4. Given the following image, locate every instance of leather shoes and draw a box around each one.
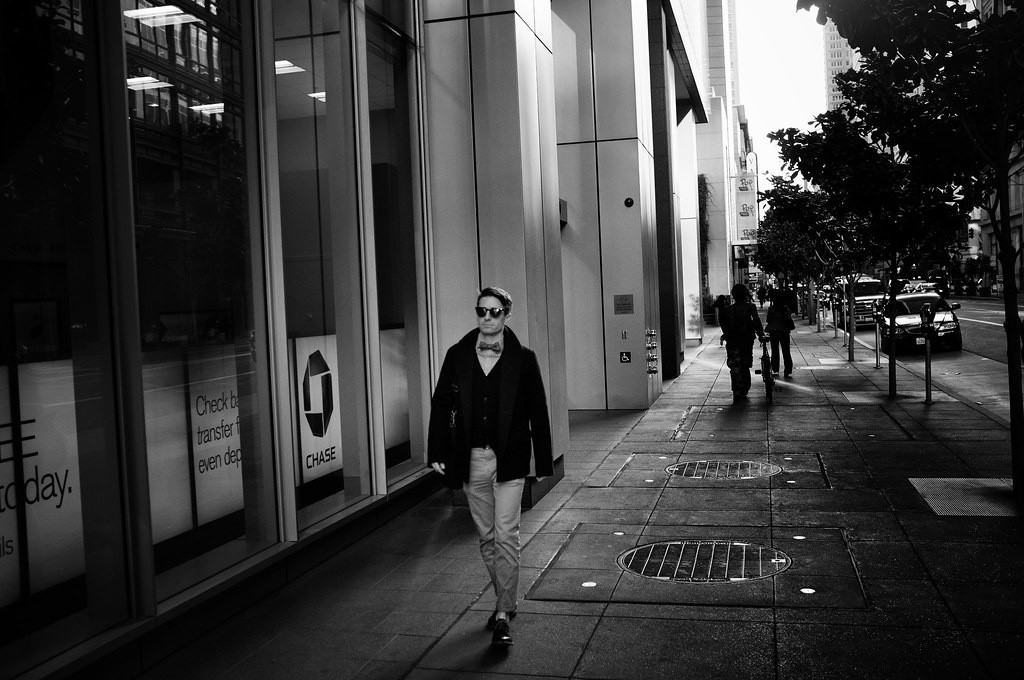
[486,609,516,630]
[492,619,513,644]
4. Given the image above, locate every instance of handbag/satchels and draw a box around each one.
[765,322,775,334]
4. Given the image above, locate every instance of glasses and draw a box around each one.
[475,306,505,318]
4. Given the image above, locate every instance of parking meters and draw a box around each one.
[821,293,827,331]
[871,299,882,369]
[831,292,839,339]
[918,302,937,405]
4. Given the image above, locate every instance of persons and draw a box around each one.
[712,282,796,400]
[427,285,555,648]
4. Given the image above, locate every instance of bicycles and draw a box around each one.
[757,332,780,410]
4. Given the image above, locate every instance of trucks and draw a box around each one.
[813,276,885,331]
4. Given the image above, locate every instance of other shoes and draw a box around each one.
[771,369,779,378]
[734,394,748,408]
[784,370,792,378]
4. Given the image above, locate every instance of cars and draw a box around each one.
[879,293,962,357]
[889,279,947,296]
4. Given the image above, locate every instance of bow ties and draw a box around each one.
[480,341,501,351]
